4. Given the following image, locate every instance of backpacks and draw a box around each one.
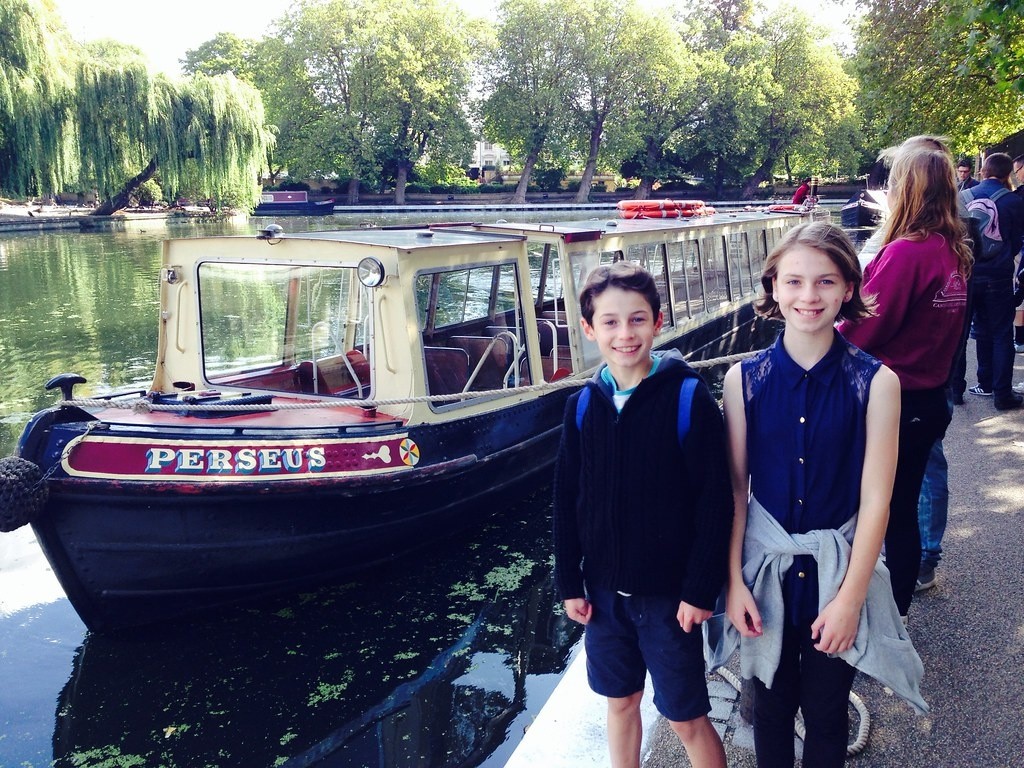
[961,188,1012,261]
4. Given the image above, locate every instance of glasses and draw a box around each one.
[1014,165,1022,175]
[958,170,969,173]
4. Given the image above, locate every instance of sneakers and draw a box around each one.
[914,569,937,593]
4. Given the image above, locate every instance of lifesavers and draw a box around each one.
[616,198,717,219]
[768,205,798,211]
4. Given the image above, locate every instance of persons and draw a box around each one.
[831,148,976,634]
[880,135,966,594]
[553,261,739,765]
[715,217,904,768]
[790,177,819,211]
[944,151,1024,414]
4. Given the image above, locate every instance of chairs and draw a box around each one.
[295,251,766,408]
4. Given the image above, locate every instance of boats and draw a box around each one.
[841,172,890,241]
[0,190,840,637]
